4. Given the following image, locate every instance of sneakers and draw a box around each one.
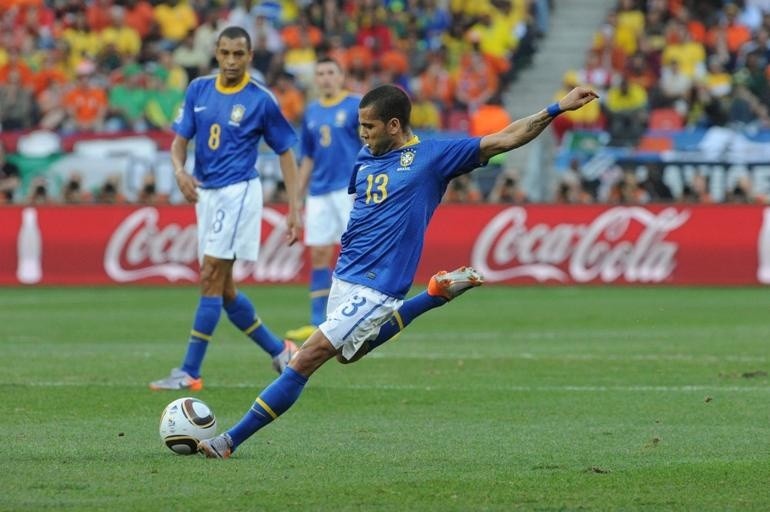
[149,368,202,390]
[428,266,485,301]
[273,339,300,374]
[198,434,234,458]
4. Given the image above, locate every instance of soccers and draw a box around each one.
[159,398,217,454]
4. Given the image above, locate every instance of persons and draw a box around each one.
[146,25,303,394]
[278,56,363,343]
[195,84,598,460]
[0,1,770,204]
